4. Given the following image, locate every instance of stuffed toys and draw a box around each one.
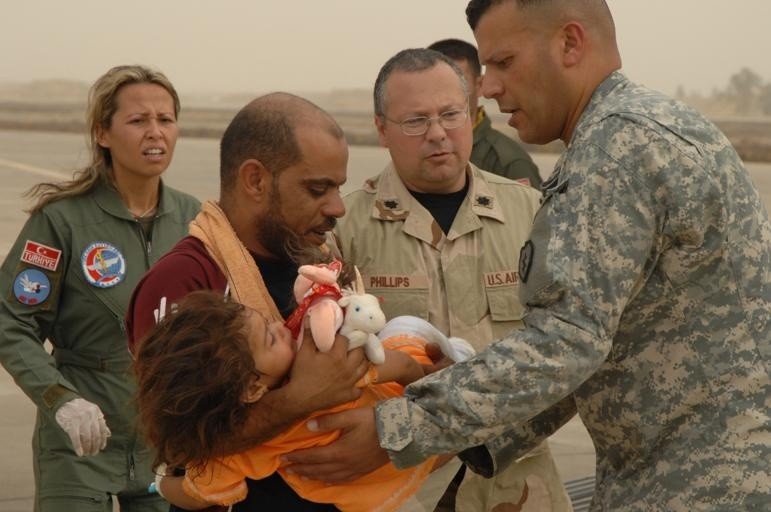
[288,258,347,355]
[336,263,389,366]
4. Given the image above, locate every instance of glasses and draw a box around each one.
[380,96,470,136]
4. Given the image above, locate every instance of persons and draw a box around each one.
[1,62,205,512]
[279,0,769,512]
[133,285,531,510]
[124,90,470,512]
[312,46,578,511]
[428,35,547,194]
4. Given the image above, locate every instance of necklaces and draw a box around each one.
[125,196,158,218]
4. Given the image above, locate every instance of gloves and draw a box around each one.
[55,398,112,456]
